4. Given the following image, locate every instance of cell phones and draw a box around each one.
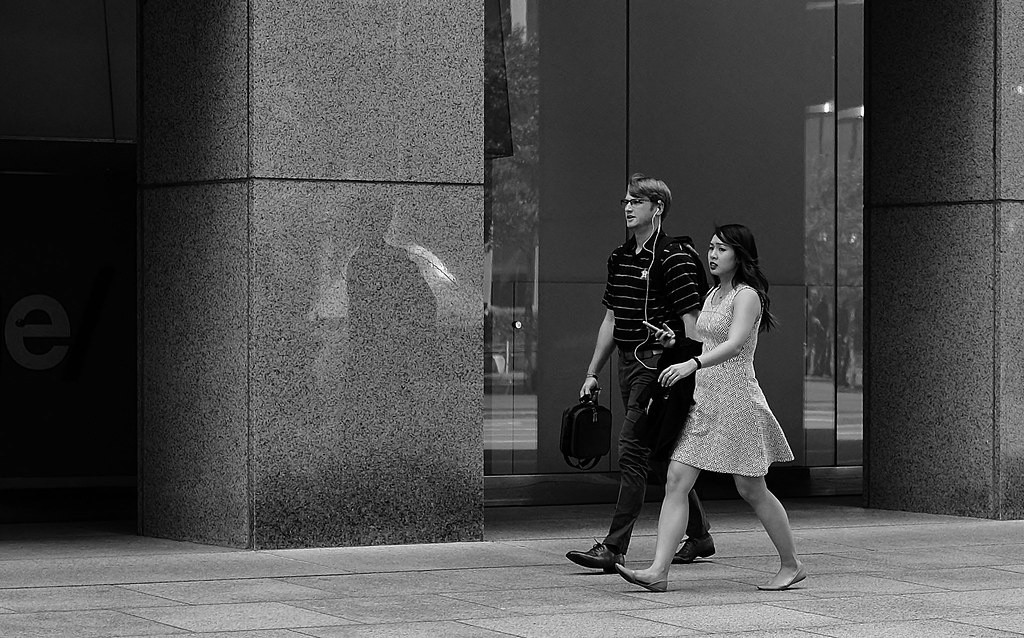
[643,321,671,341]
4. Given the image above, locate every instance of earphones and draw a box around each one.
[655,208,660,213]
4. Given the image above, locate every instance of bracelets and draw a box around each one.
[692,357,701,370]
[586,373,598,380]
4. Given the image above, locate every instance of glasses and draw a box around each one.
[620,199,650,208]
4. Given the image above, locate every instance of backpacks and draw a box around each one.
[655,236,709,310]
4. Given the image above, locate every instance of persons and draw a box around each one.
[615,223,807,592]
[565,172,715,574]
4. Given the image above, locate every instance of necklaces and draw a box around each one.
[719,289,732,298]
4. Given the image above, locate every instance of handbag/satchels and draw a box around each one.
[559,396,612,470]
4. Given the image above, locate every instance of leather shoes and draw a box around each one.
[672,535,715,563]
[566,538,625,573]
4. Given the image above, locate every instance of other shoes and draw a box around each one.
[757,565,807,590]
[615,562,668,592]
